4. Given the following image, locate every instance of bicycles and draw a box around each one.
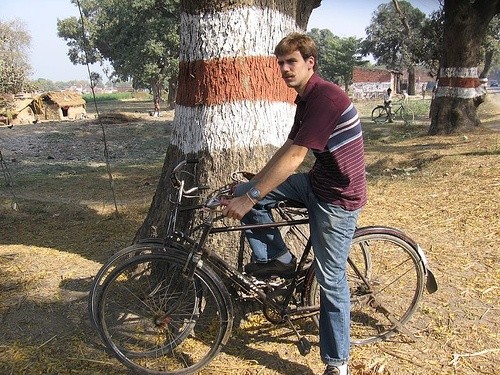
[87,159,372,358]
[371,96,415,125]
[96,188,438,372]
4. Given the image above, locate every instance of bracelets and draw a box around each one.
[245,192,256,206]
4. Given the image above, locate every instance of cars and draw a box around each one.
[491,83,498,87]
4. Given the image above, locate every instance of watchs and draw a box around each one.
[248,186,262,202]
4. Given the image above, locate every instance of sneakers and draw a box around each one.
[322,363,351,375]
[245,249,297,278]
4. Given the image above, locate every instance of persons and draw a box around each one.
[218,33,366,375]
[153,95,160,117]
[422,84,426,100]
[384,88,395,123]
